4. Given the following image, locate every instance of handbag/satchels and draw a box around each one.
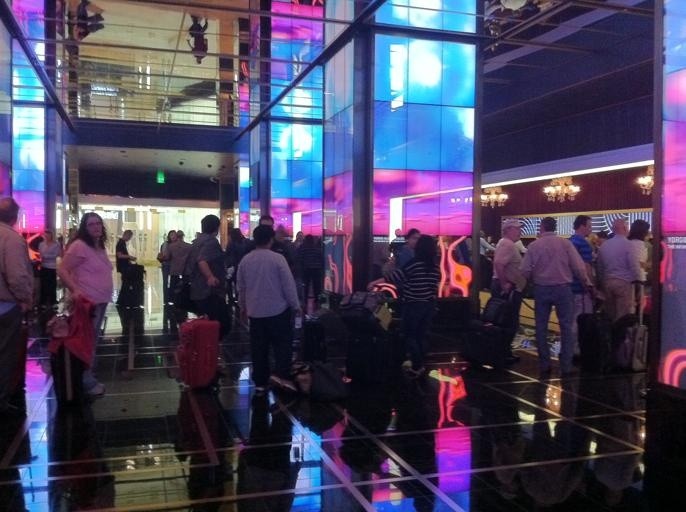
[340,290,382,320]
[48,314,75,338]
[201,295,232,338]
[172,277,191,309]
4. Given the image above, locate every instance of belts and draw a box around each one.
[538,283,569,287]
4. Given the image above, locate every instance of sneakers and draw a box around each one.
[83,383,104,395]
[503,354,519,363]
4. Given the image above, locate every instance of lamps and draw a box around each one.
[634,163,656,196]
[480,187,508,208]
[542,177,580,201]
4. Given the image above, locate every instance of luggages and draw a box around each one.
[178,318,220,390]
[281,314,326,366]
[616,280,649,371]
[577,284,609,362]
[122,265,144,307]
[52,296,95,404]
[468,290,515,381]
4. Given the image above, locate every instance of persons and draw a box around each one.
[159,230,193,340]
[37,229,59,309]
[192,215,233,342]
[246,215,293,269]
[490,218,528,366]
[275,226,326,314]
[584,230,616,258]
[237,391,305,512]
[187,15,208,64]
[0,198,37,412]
[629,219,650,323]
[463,374,643,512]
[596,219,647,323]
[116,230,137,304]
[57,213,113,396]
[226,227,255,263]
[395,229,421,269]
[325,236,338,271]
[479,230,496,289]
[156,230,178,304]
[561,215,594,358]
[366,235,441,378]
[236,225,304,391]
[338,376,441,512]
[519,217,594,375]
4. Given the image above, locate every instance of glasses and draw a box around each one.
[82,221,103,226]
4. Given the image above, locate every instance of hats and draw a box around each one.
[502,218,524,229]
